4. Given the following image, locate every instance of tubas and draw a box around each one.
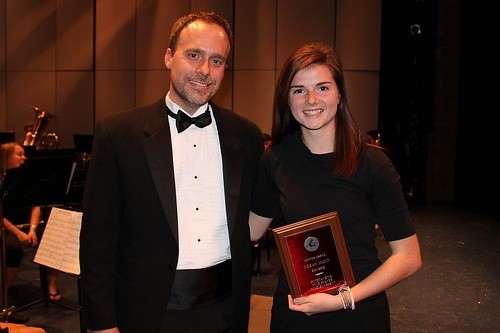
[22,104,59,151]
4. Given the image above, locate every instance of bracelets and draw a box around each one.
[30,225,38,230]
[337,285,355,310]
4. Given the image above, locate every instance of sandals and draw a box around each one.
[49,289,62,301]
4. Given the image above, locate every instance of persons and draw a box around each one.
[0,142,61,306]
[79,12,378,333]
[261,131,273,153]
[248,40,423,333]
[364,129,388,153]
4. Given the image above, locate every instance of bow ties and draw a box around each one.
[166,106,212,134]
